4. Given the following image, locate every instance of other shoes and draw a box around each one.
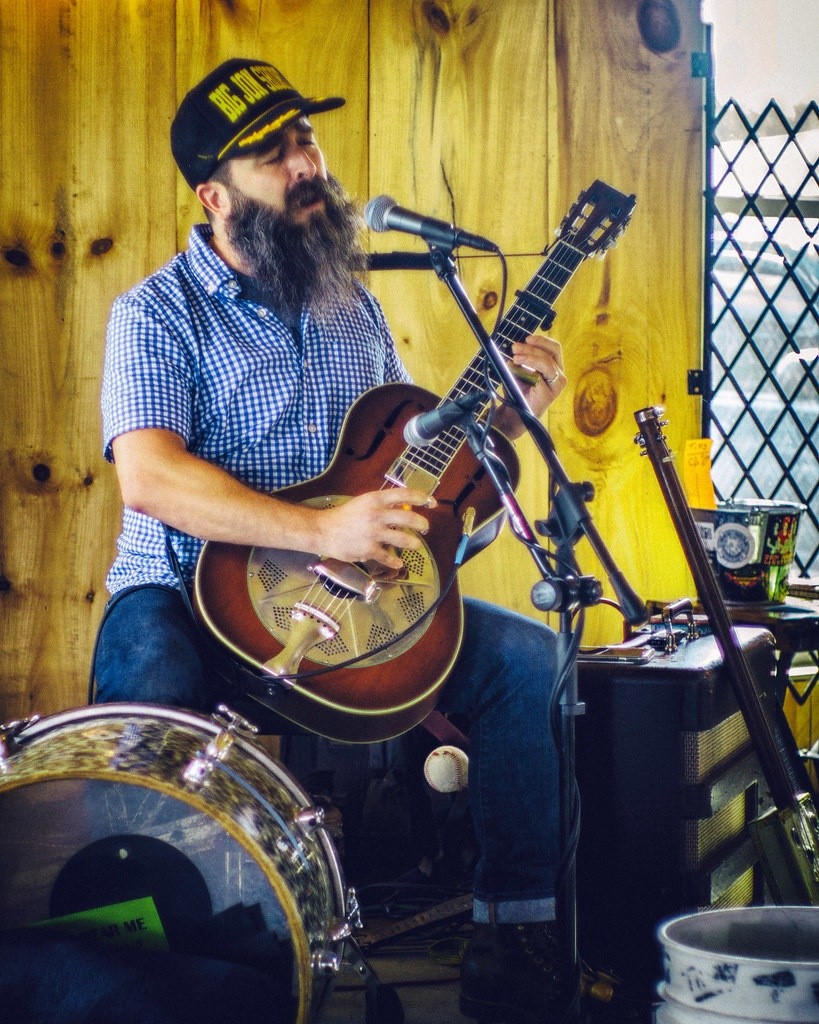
[457,922,635,1022]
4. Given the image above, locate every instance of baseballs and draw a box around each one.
[423,745,469,794]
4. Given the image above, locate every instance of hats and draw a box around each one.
[170,58,346,193]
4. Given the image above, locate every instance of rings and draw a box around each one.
[547,372,559,383]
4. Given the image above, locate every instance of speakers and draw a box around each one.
[574,622,772,966]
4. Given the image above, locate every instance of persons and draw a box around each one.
[93,58,653,1024]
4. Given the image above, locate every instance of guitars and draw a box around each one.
[190,180,639,743]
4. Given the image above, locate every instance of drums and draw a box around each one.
[1,704,352,1024]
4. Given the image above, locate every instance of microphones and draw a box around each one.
[403,388,484,447]
[363,194,499,254]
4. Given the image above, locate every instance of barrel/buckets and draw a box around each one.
[690,498,808,606]
[655,906,819,1024]
